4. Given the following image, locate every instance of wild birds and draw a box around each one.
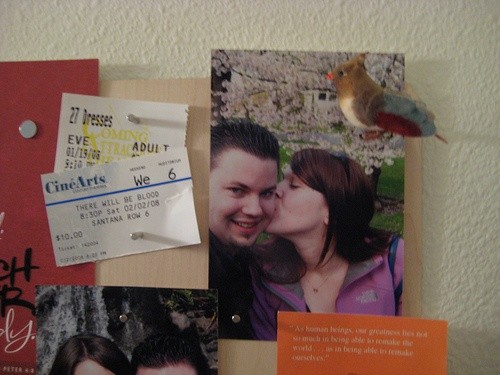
[325,51,437,141]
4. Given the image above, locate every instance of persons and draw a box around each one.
[248,145,403,340]
[47,333,133,375]
[133,326,216,375]
[24,120,280,375]
